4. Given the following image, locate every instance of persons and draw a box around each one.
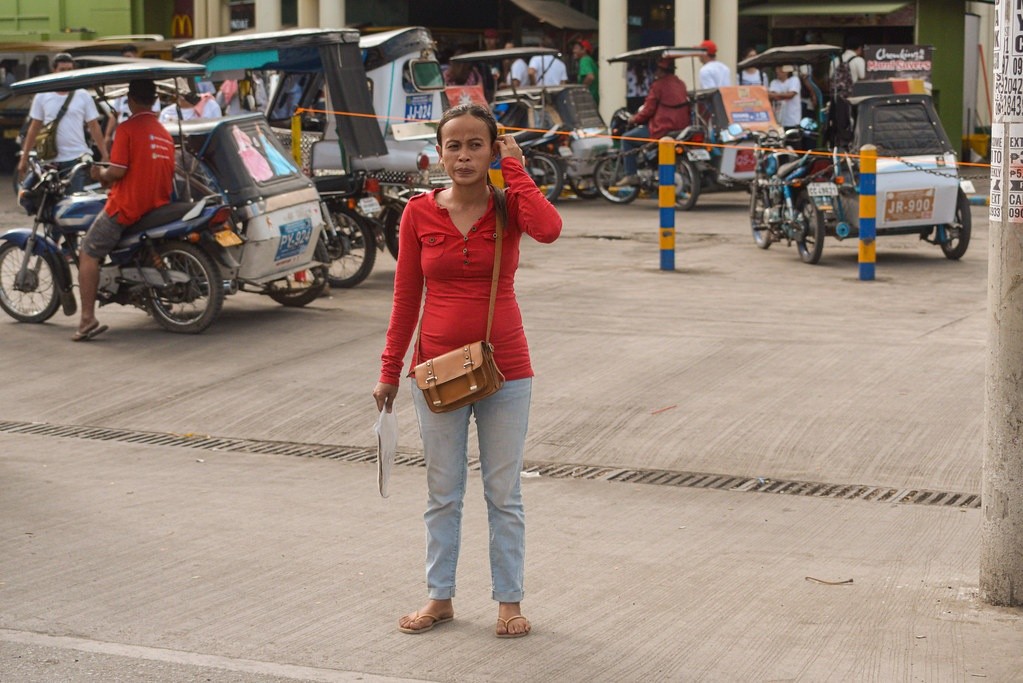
[373,104,564,639]
[444,38,602,107]
[69,77,177,344]
[18,55,117,195]
[617,59,693,189]
[699,41,867,149]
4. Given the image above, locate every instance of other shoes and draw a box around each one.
[615,176,641,186]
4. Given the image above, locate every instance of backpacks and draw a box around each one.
[829,54,858,102]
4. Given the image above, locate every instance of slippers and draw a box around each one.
[398,609,453,634]
[71,325,107,342]
[496,615,529,637]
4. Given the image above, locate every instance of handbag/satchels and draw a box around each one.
[35,129,58,161]
[414,341,506,413]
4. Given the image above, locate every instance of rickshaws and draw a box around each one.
[0,33,166,175]
[169,26,457,289]
[0,57,334,334]
[438,45,615,204]
[736,42,972,265]
[594,44,787,210]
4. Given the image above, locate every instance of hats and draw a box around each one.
[700,40,717,56]
[484,29,498,38]
[576,38,592,53]
